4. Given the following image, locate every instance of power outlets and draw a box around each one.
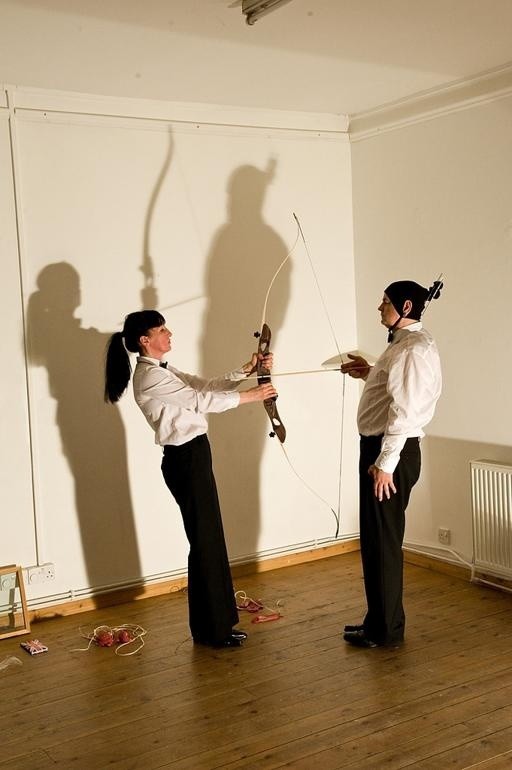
[40,563,56,585]
[438,528,451,545]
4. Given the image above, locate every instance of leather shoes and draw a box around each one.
[205,637,241,646]
[230,631,246,641]
[342,624,364,632]
[343,630,378,647]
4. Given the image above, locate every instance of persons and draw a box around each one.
[340,281,443,648]
[103,310,278,646]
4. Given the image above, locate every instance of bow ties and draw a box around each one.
[387,331,394,343]
[160,361,169,370]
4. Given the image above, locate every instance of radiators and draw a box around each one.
[468,458,512,581]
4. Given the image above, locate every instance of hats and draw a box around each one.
[385,281,443,320]
